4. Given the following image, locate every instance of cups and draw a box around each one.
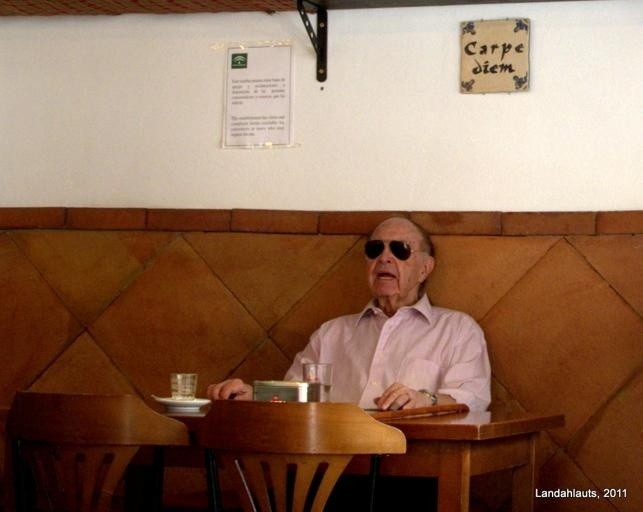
[303,363,334,403]
[170,372,198,401]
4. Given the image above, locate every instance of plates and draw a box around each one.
[150,394,212,413]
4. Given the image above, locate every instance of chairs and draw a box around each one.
[9,390,407,511]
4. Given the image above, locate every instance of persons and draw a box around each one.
[207,216,492,512]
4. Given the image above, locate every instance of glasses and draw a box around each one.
[364,239,430,261]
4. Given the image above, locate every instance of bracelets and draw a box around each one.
[419,389,438,405]
